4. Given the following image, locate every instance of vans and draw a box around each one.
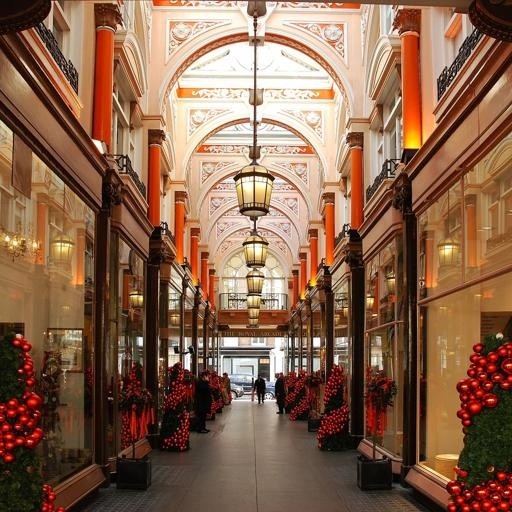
[227,373,258,395]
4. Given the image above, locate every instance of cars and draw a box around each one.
[264,381,278,400]
[230,382,244,400]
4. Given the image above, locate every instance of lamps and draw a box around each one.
[235,0,281,217]
[169,312,181,328]
[384,255,398,297]
[241,216,270,327]
[333,258,379,328]
[0,225,40,266]
[436,185,464,271]
[128,289,145,312]
[51,182,75,270]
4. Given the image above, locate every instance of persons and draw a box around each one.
[222,372,232,403]
[280,372,285,382]
[252,373,267,405]
[273,373,286,415]
[194,372,210,434]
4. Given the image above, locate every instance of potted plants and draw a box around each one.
[284,367,324,434]
[319,365,350,451]
[112,365,162,497]
[351,360,402,492]
[144,362,224,452]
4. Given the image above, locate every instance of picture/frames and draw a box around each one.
[43,325,83,376]
